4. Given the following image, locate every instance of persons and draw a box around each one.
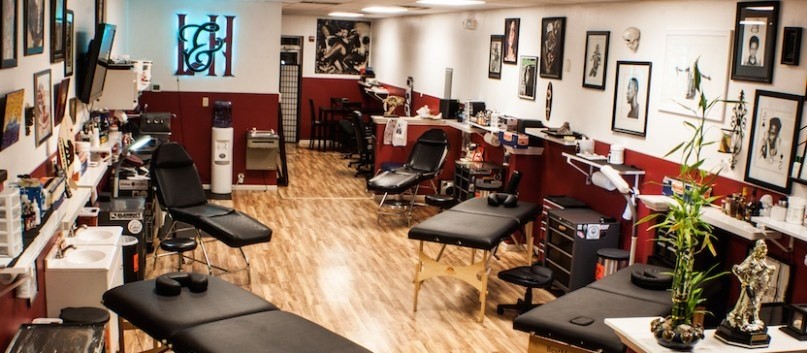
[491,42,501,72]
[761,117,783,159]
[524,64,534,97]
[544,20,562,75]
[503,19,516,62]
[37,84,51,141]
[316,18,369,75]
[623,27,640,51]
[626,77,639,119]
[675,56,712,99]
[743,36,762,67]
[726,239,776,332]
[589,44,601,77]
[57,90,66,121]
[3,98,20,147]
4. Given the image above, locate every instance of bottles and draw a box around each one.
[21,194,41,231]
[475,109,494,126]
[457,107,463,122]
[116,59,153,86]
[75,117,132,168]
[721,185,762,228]
[214,101,232,128]
[791,156,802,179]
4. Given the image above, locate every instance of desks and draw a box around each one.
[513,262,678,353]
[562,152,646,212]
[100,271,371,353]
[667,201,783,244]
[406,192,544,324]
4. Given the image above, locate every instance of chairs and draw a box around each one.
[307,96,362,151]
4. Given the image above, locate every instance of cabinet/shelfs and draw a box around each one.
[538,195,588,261]
[545,208,621,299]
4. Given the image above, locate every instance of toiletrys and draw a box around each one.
[475,109,492,126]
[81,120,122,165]
[719,185,786,231]
[11,173,42,231]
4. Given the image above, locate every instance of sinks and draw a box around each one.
[45,224,123,271]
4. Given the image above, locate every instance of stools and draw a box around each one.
[58,305,110,327]
[595,248,630,281]
[497,262,555,318]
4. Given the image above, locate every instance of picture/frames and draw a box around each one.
[487,16,653,135]
[1,1,78,153]
[732,1,783,83]
[742,88,807,196]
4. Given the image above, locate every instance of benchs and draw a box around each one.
[149,141,272,275]
[367,129,450,228]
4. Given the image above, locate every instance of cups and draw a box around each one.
[607,146,625,165]
[581,140,595,155]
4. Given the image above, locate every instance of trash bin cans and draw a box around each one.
[595,248,629,281]
[475,182,501,198]
[122,236,140,283]
[60,306,112,353]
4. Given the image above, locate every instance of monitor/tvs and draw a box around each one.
[77,22,117,104]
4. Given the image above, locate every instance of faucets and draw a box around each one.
[55,238,77,260]
[67,222,88,240]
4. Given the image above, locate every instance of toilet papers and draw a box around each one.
[483,132,501,146]
[592,171,617,191]
